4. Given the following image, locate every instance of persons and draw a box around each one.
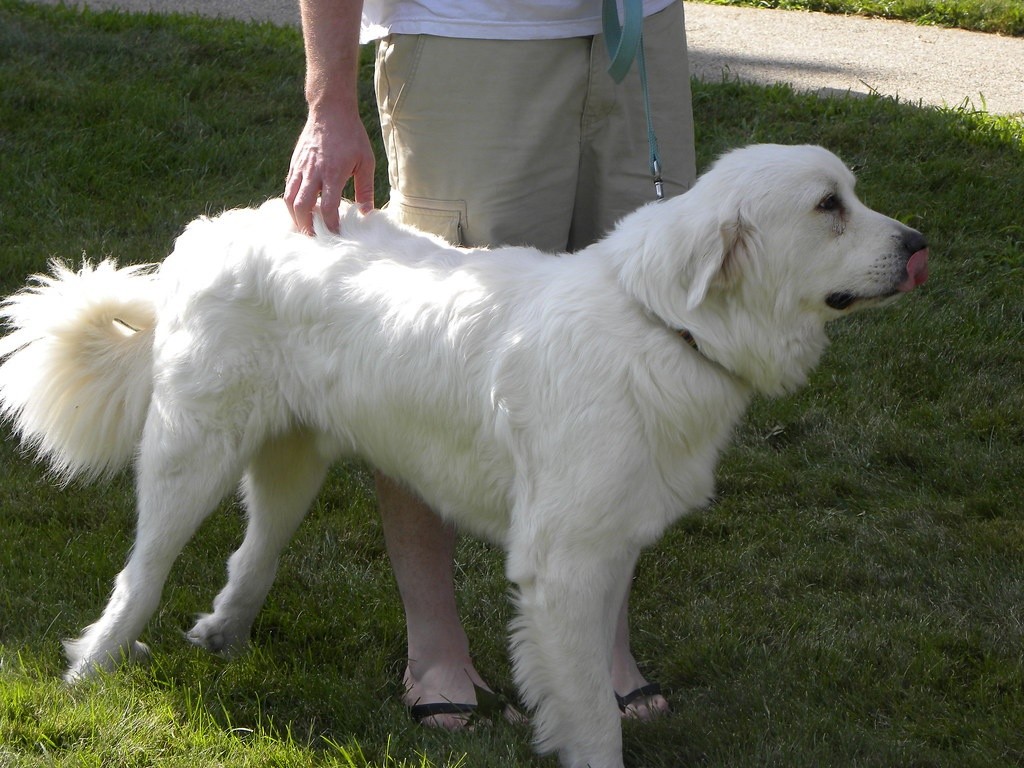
[286,1,697,731]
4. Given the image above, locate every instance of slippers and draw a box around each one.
[614,685,664,722]
[413,694,512,735]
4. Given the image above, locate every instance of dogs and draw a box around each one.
[0,143,930,768]
